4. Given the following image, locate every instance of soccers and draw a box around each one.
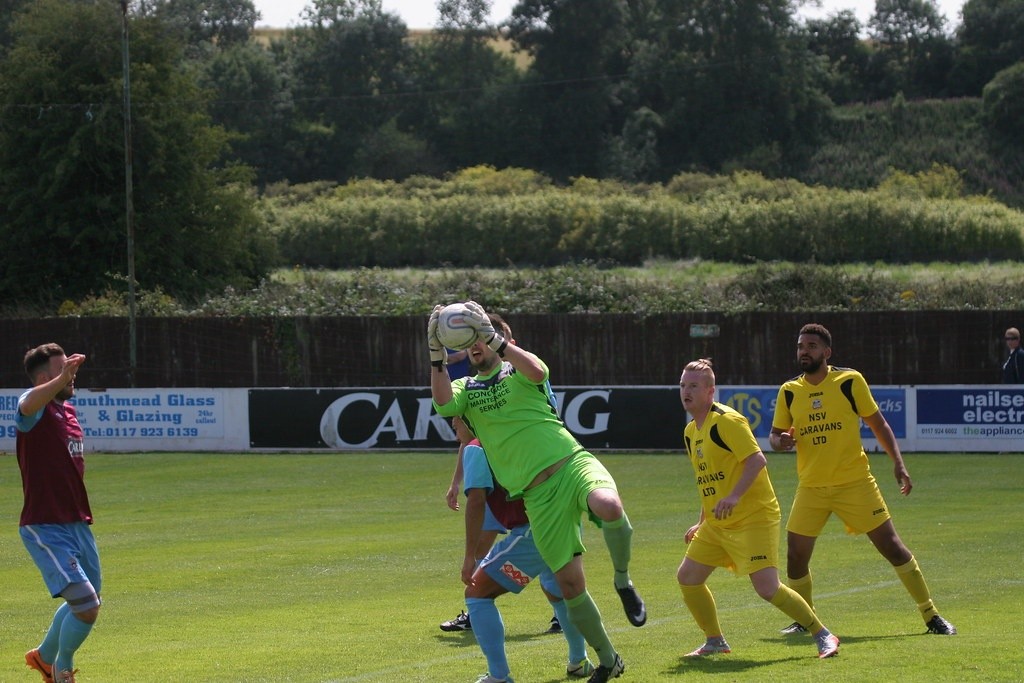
[435,303,479,352]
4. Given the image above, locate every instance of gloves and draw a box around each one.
[426,304,449,365]
[462,300,506,352]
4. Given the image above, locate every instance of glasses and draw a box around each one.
[1005,337,1017,341]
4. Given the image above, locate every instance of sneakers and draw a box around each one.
[587,652,624,683]
[440,610,473,631]
[614,580,646,627]
[925,615,956,634]
[548,617,563,632]
[814,626,840,658]
[51,664,80,683]
[781,621,810,634]
[25,649,55,683]
[475,672,514,683]
[567,658,594,676]
[684,635,731,657]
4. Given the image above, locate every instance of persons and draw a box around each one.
[998,328,1024,384]
[439,415,594,683]
[14,343,101,683]
[769,323,956,636]
[428,301,647,683]
[675,359,840,660]
